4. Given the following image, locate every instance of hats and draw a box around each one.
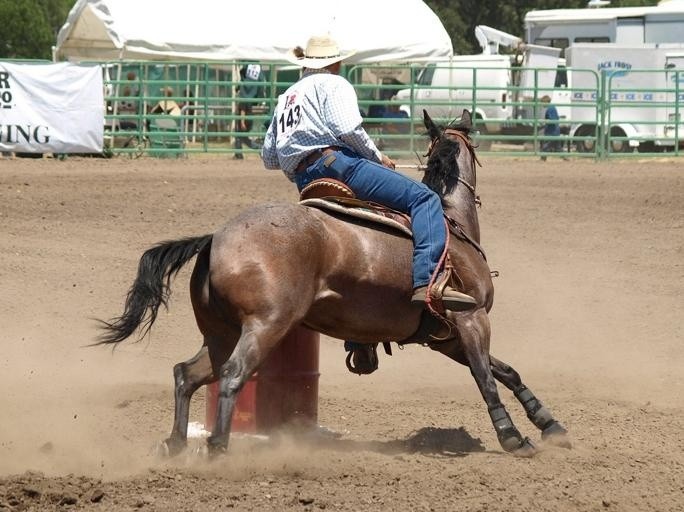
[283,35,357,69]
[159,86,173,96]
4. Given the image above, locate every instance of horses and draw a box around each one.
[76,107,576,461]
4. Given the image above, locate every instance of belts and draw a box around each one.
[294,146,341,171]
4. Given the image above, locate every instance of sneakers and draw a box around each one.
[409,282,478,312]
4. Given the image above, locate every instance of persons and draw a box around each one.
[182,90,197,125]
[236,60,267,131]
[534,95,567,162]
[262,32,476,374]
[123,73,140,96]
[382,95,416,142]
[153,88,181,118]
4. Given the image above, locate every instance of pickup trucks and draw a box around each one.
[394,52,572,151]
